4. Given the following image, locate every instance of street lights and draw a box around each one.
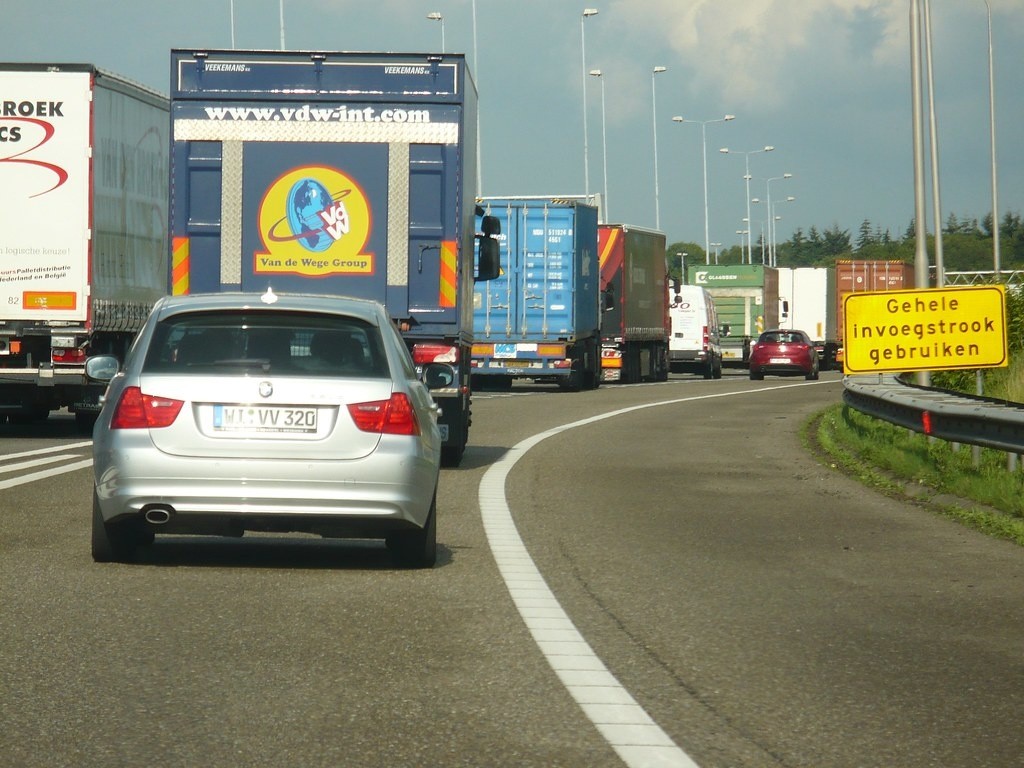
[589,68,609,223]
[676,252,688,284]
[426,12,445,52]
[673,114,735,266]
[720,145,794,268]
[652,65,668,229]
[710,242,724,267]
[579,6,599,203]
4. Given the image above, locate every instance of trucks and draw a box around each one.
[773,265,837,370]
[1,61,172,429]
[687,262,791,369]
[471,195,619,395]
[835,257,915,340]
[167,48,504,470]
[597,222,683,385]
[669,284,733,377]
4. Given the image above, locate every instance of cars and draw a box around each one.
[747,328,820,381]
[835,342,844,372]
[83,280,446,567]
